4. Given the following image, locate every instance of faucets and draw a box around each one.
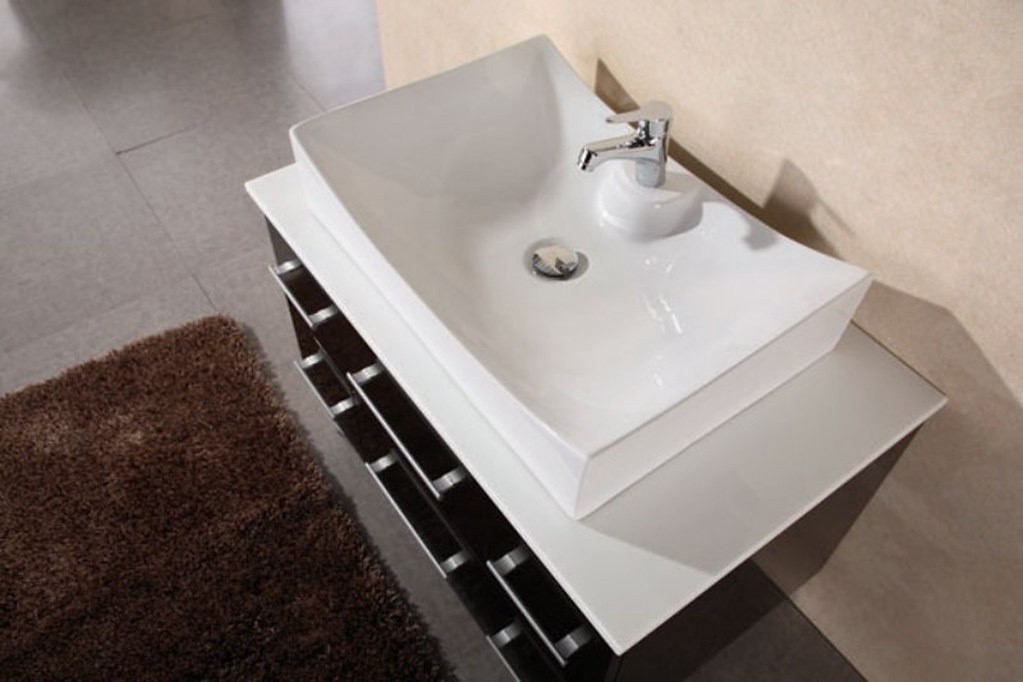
[577,102,675,186]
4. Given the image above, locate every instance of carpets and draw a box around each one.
[0,317,455,682]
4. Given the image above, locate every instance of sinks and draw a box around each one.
[289,35,874,522]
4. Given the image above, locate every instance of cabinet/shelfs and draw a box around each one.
[267,216,622,682]
